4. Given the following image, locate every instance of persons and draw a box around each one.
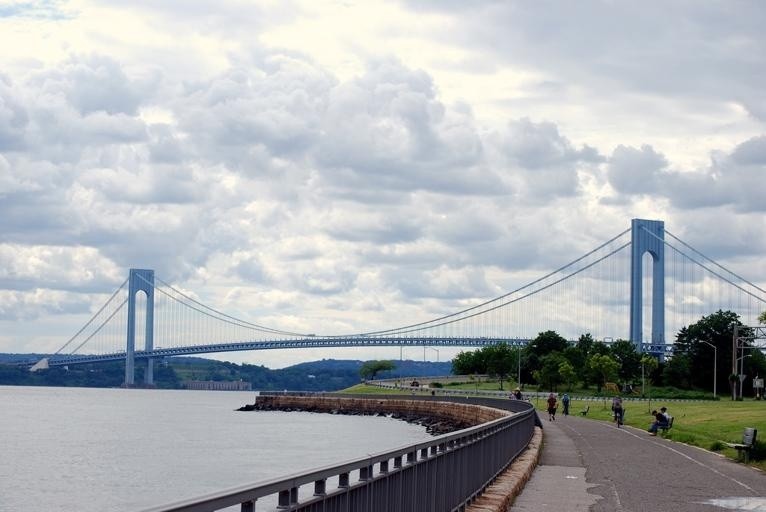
[546,393,559,421]
[649,406,669,433]
[508,390,517,400]
[611,393,624,425]
[649,410,669,436]
[561,393,569,417]
[513,386,522,399]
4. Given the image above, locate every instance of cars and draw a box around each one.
[409,381,419,387]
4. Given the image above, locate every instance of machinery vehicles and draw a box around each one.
[605,382,641,398]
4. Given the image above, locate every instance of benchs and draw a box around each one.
[579,406,590,415]
[726,426,757,464]
[658,417,674,433]
[612,408,625,420]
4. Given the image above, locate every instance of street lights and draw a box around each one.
[732,354,752,401]
[697,340,716,399]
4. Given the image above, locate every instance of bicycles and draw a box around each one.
[613,411,621,428]
